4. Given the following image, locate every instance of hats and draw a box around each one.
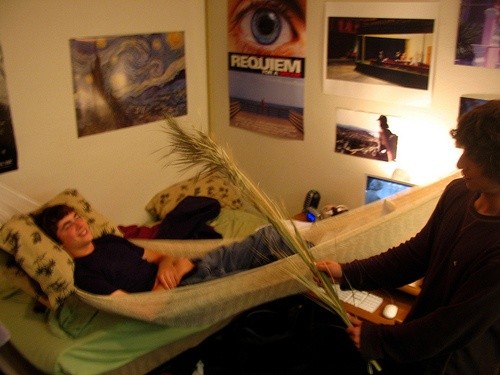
[378,115,386,120]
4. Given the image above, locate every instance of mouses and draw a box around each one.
[383,304,397,319]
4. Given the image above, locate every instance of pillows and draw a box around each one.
[147,170,245,220]
[0,189,125,305]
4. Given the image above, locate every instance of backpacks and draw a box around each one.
[389,134,398,159]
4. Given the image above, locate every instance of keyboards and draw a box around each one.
[319,284,382,314]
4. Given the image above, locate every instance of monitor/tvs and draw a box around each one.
[364,173,415,203]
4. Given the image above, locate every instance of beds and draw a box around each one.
[0,208,271,375]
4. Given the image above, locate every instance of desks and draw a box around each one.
[288,209,421,328]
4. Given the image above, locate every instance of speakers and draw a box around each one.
[303,189,320,212]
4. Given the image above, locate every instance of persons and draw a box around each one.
[378,48,418,66]
[31,203,315,322]
[310,100,500,375]
[227,0,306,57]
[372,114,398,162]
